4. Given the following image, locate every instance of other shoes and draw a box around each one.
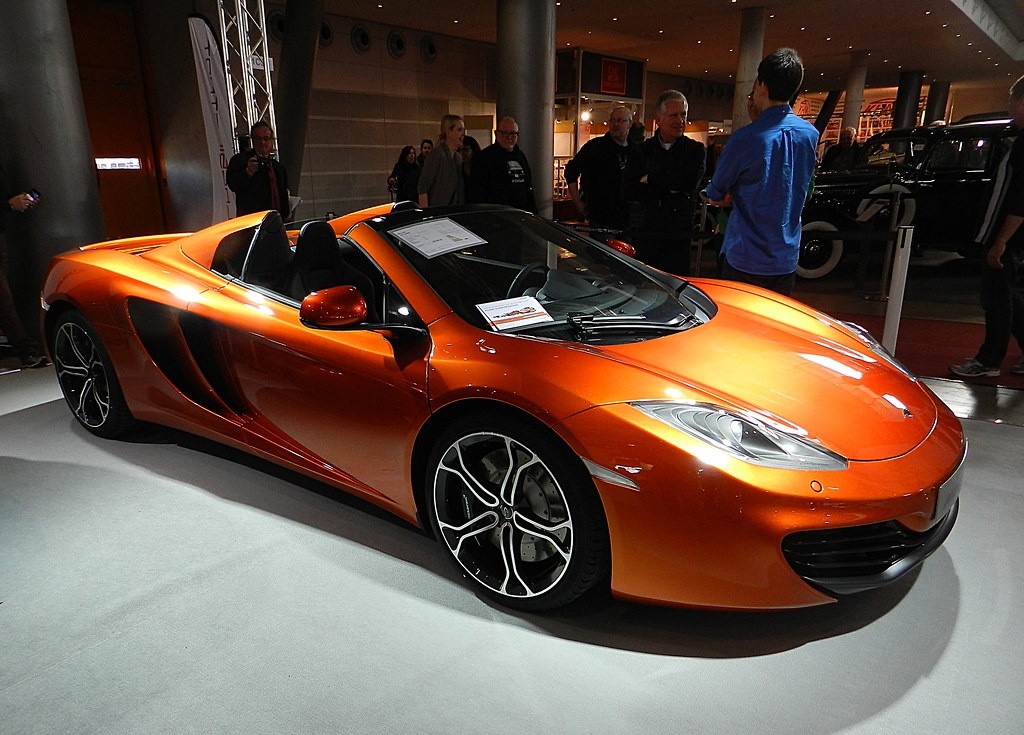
[24,355,47,368]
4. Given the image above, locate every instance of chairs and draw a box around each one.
[939,141,960,168]
[388,200,494,327]
[287,221,381,324]
[916,140,944,168]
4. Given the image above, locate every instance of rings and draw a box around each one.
[992,258,997,262]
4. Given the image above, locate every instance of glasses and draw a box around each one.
[747,94,753,100]
[253,137,271,142]
[497,131,520,136]
[608,118,629,123]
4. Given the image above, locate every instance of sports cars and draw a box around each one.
[39,199,968,616]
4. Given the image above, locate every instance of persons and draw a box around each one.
[417,115,466,209]
[0,191,47,369]
[226,121,290,223]
[948,73,1024,378]
[387,145,421,204]
[465,116,538,215]
[822,127,868,171]
[620,89,706,274]
[415,139,434,167]
[707,48,820,296]
[456,136,481,178]
[565,106,636,243]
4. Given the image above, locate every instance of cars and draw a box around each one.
[690,113,1013,285]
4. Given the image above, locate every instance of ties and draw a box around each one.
[269,160,280,212]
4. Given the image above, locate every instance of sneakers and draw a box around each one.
[1010,364,1024,374]
[950,357,1000,377]
